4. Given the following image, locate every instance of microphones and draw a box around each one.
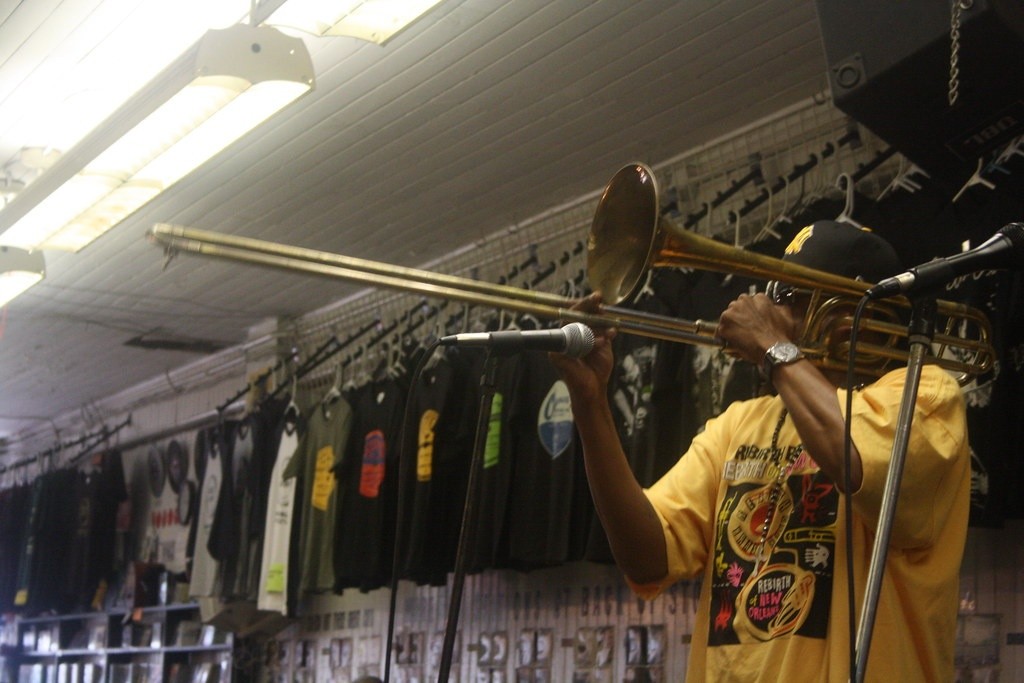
[440,323,595,357]
[866,221,1024,302]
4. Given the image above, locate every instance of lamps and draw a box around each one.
[0,0,446,314]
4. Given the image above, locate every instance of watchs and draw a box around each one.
[760,342,805,398]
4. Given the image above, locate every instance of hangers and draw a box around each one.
[231,126,1024,430]
[0,415,127,497]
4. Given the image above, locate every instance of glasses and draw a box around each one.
[773,282,815,306]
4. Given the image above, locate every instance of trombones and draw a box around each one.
[152,164,994,386]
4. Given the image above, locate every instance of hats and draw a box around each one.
[787,221,904,284]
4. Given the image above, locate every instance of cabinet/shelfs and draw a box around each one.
[14,571,254,683]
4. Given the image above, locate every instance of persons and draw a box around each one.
[557,218,971,683]
